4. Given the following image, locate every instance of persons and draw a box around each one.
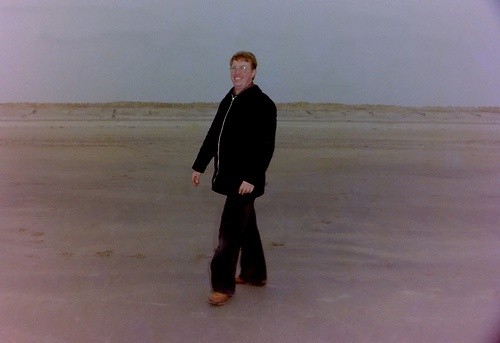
[192,51,277,305]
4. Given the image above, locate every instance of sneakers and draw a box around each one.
[234,274,265,284]
[206,290,233,305]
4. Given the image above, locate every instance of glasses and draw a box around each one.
[230,65,253,72]
